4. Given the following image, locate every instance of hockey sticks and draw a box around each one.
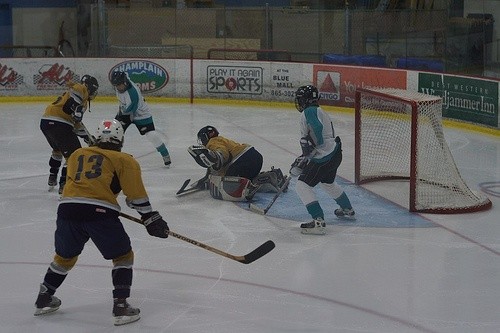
[248,174,293,215]
[80,121,95,144]
[176,179,198,195]
[120,212,275,265]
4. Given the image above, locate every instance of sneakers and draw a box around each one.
[58,176,67,200]
[163,154,171,168]
[270,166,287,191]
[334,207,355,219]
[34,284,61,315]
[112,301,141,325]
[301,217,325,235]
[47,173,57,191]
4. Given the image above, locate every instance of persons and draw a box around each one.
[110,72,172,167]
[34,119,170,325]
[187,126,288,203]
[40,74,99,195]
[289,85,355,235]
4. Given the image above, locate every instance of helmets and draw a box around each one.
[294,86,319,112]
[196,126,219,147]
[96,119,123,151]
[81,75,99,96]
[111,71,129,94]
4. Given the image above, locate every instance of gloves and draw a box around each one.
[141,210,169,238]
[85,135,96,146]
[289,154,309,177]
[205,166,217,177]
[72,112,83,122]
